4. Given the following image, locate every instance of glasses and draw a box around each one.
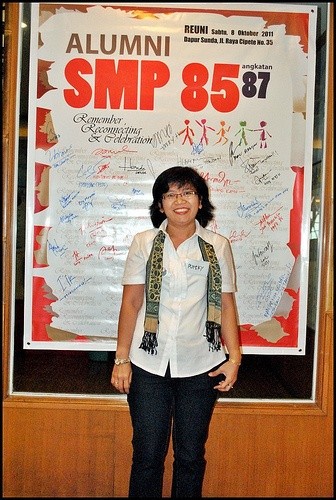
[161,191,199,200]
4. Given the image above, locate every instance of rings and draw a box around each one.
[228,383,234,389]
[114,381,119,385]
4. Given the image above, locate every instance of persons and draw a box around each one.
[111,167,242,500]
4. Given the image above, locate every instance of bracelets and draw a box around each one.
[114,358,131,365]
[228,359,242,365]
